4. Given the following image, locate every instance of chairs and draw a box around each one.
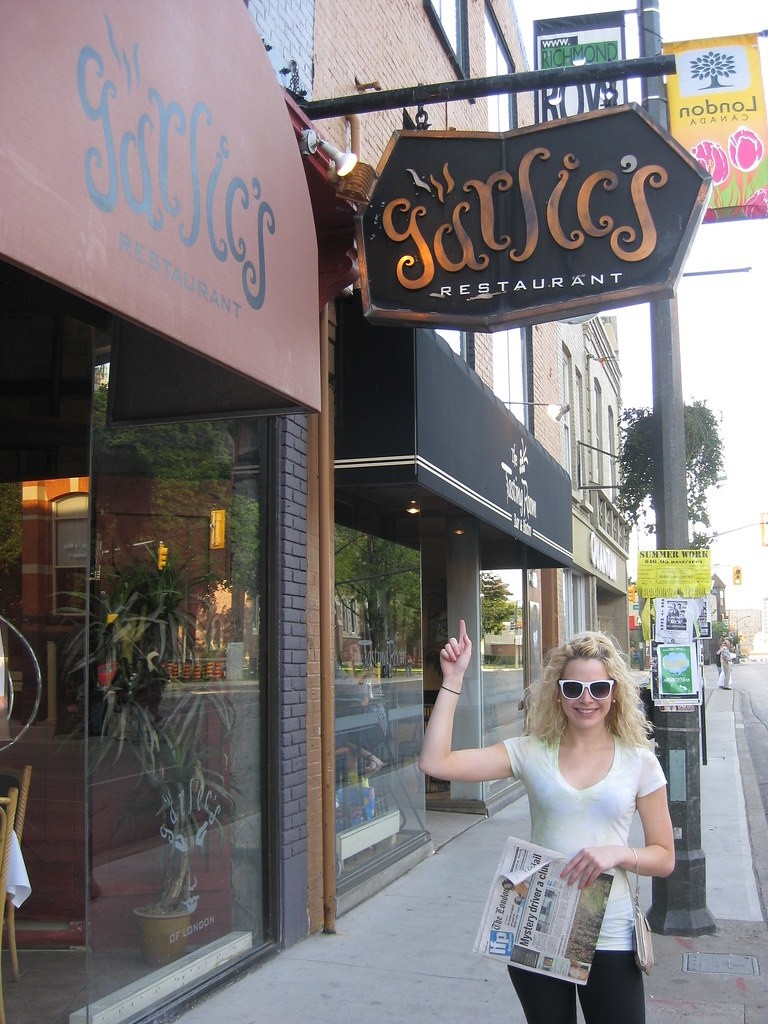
[0,786,19,1024]
[398,690,450,795]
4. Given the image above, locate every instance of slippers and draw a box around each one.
[363,754,388,778]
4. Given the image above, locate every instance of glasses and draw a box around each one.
[556,679,615,700]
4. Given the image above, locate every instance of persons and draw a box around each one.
[404,653,414,680]
[104,640,169,744]
[713,639,736,689]
[666,603,686,626]
[419,618,676,1024]
[336,638,387,775]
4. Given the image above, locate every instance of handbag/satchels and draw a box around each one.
[717,671,732,686]
[625,848,654,975]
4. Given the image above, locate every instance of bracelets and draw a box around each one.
[440,685,462,696]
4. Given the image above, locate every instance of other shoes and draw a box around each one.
[724,688,731,690]
[335,803,361,823]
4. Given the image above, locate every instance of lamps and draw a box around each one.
[503,402,571,421]
[316,139,357,177]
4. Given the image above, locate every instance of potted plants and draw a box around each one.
[45,539,247,970]
